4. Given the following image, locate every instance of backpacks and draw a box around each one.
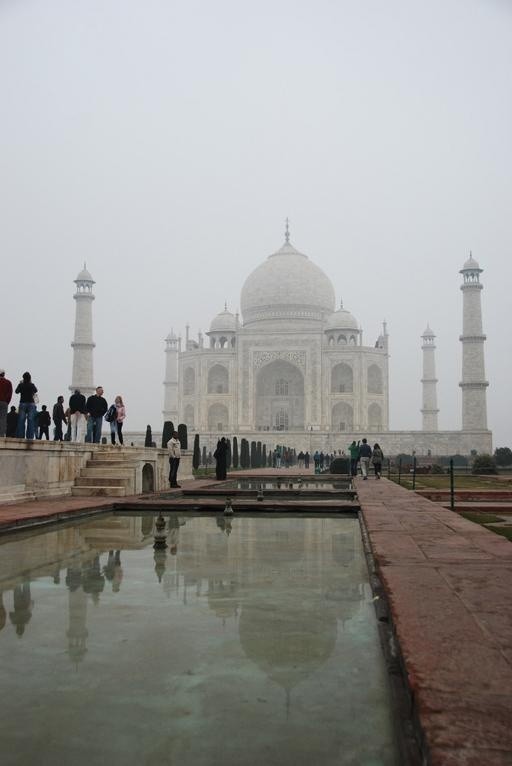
[105,406,116,423]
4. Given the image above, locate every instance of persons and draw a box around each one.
[26,405,39,438]
[38,404,50,440]
[107,549,124,593]
[166,430,183,487]
[65,564,81,594]
[53,395,68,440]
[0,591,7,631]
[8,575,33,639]
[166,513,181,556]
[82,555,104,605]
[68,387,86,443]
[0,370,13,436]
[108,394,125,446]
[50,569,61,584]
[86,385,108,443]
[6,405,19,437]
[270,436,384,481]
[64,402,71,441]
[213,434,230,481]
[15,372,37,438]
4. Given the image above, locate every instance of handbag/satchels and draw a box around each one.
[33,393,40,404]
